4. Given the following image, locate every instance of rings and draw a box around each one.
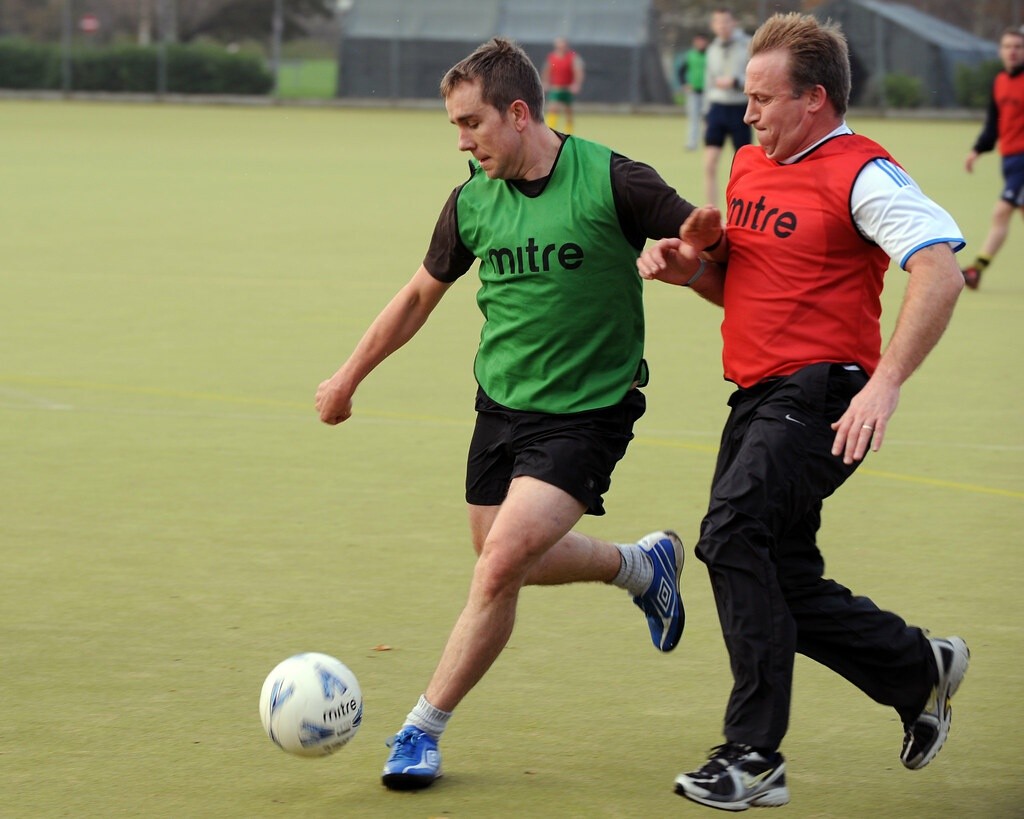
[862,424,874,432]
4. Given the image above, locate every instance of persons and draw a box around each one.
[540,35,585,135]
[676,33,707,150]
[635,8,972,812]
[315,34,731,790]
[960,28,1024,288]
[698,8,754,208]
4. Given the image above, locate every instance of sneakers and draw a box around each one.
[672,743,791,811]
[960,269,981,289]
[632,530,686,651]
[900,630,970,771]
[380,727,441,789]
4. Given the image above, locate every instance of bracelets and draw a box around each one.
[681,256,707,288]
[701,228,724,254]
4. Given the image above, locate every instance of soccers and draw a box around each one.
[257,651,365,760]
